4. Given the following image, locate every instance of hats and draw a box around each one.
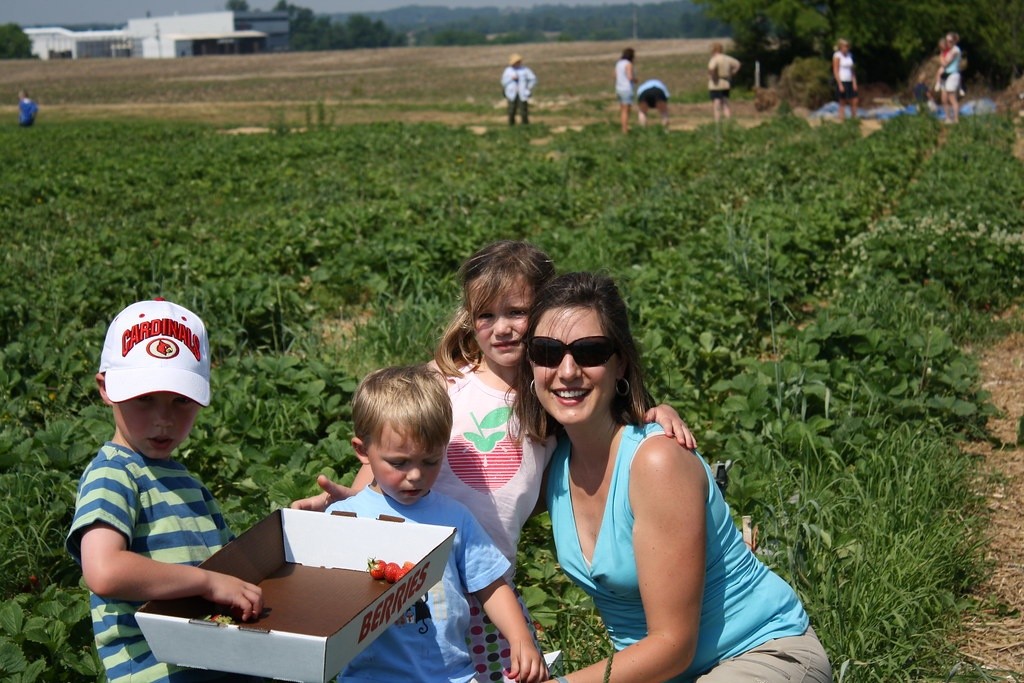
[98,297,211,408]
[510,53,522,65]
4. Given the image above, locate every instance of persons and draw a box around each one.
[500,53,537,127]
[833,38,858,119]
[636,79,670,135]
[708,43,741,121]
[912,74,932,111]
[615,48,639,134]
[65,298,264,683]
[291,239,833,683]
[17,88,38,127]
[934,32,965,124]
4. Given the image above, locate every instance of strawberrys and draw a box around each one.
[369,560,416,583]
[210,609,244,625]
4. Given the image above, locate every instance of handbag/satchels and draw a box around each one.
[941,71,952,80]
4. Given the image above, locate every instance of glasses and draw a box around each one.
[523,334,617,368]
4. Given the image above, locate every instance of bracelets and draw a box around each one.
[555,677,568,683]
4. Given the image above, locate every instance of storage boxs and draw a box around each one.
[134,508,457,683]
